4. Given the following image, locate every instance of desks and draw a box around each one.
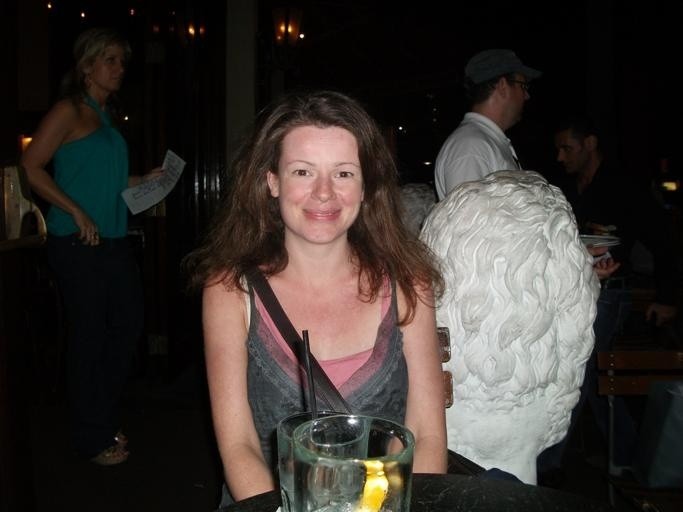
[212,473,601,511]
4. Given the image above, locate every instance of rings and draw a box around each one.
[91,231,97,242]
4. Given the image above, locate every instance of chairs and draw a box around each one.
[595,346,683,511]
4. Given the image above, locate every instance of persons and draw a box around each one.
[555,118,629,231]
[18,25,166,469]
[418,172,602,485]
[433,42,620,464]
[178,90,449,512]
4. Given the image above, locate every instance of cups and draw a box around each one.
[290,414,412,511]
[275,416,366,510]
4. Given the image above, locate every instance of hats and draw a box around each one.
[464,48,543,85]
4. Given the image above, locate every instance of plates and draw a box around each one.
[575,233,620,247]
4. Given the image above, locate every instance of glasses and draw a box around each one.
[509,80,530,90]
